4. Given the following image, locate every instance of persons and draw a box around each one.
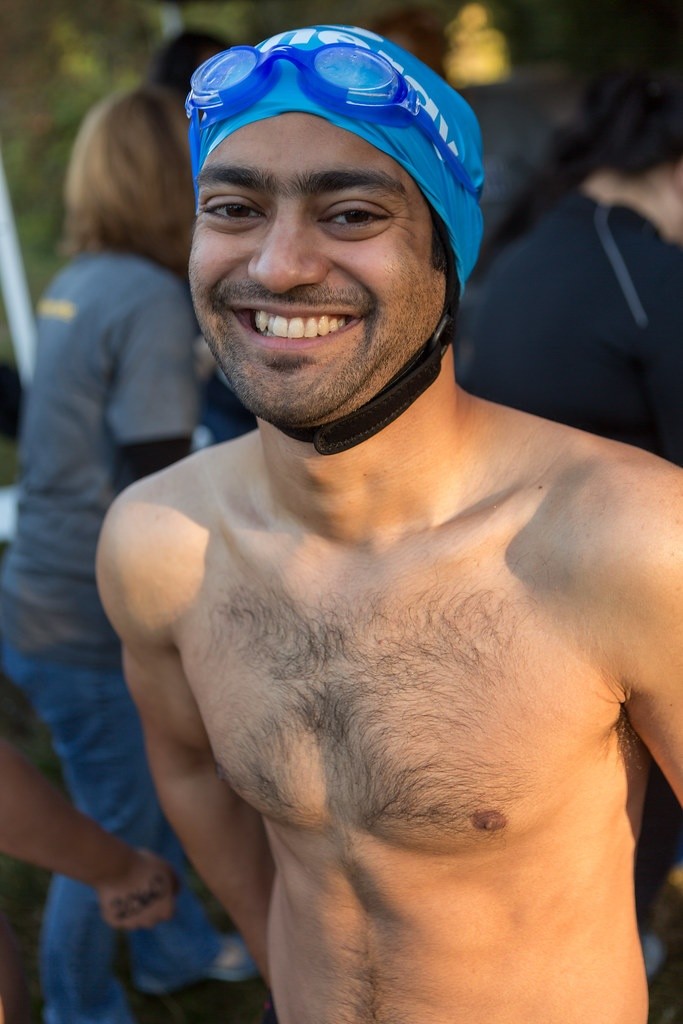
[150,28,242,95]
[96,25,683,1024]
[0,84,277,1024]
[1,659,180,1024]
[450,41,682,980]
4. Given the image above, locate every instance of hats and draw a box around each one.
[198,25,483,297]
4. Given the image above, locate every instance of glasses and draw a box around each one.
[185,43,481,212]
